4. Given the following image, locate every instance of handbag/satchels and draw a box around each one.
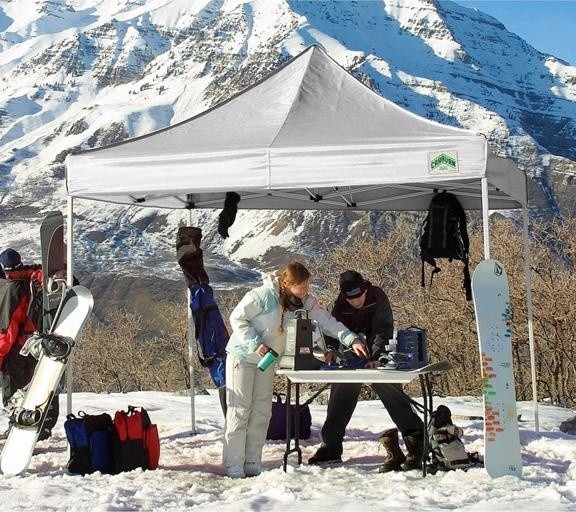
[266,402,312,440]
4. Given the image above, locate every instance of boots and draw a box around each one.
[377,428,407,473]
[400,431,428,471]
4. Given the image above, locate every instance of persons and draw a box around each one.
[221,264,369,480]
[307,270,429,471]
[0,249,59,441]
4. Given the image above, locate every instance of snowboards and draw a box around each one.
[471,258,524,478]
[0,211,94,476]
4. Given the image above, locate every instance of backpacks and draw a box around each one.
[419,190,469,260]
[427,405,472,471]
[26,268,80,334]
[64,405,160,476]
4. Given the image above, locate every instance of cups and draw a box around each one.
[256,347,280,372]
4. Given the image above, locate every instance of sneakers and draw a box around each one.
[308,442,343,467]
[225,463,261,477]
[0,431,9,444]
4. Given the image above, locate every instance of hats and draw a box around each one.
[339,269,366,299]
[0,248,21,270]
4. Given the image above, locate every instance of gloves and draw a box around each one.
[218,192,240,239]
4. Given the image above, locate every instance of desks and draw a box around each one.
[276,369,437,477]
